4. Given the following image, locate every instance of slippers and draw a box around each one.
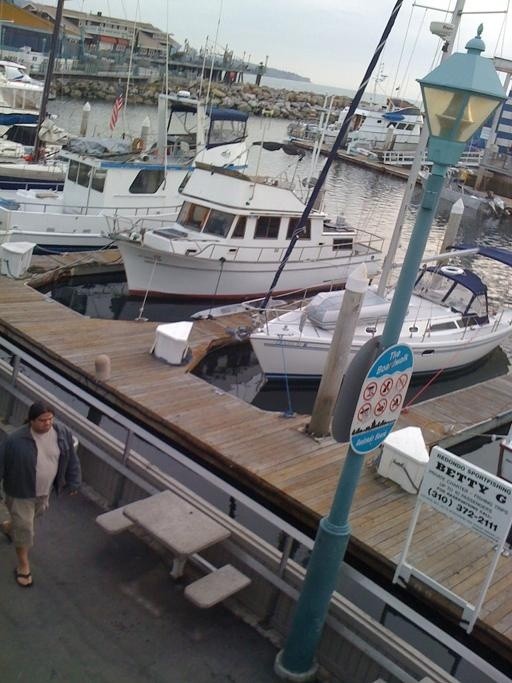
[15,567,33,588]
[0,522,12,541]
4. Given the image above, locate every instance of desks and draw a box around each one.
[122,489,233,581]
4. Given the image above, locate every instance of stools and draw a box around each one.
[183,564,253,609]
[95,501,137,536]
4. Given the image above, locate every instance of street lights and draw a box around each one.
[270,20,509,682]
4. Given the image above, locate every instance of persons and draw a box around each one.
[0,400,82,587]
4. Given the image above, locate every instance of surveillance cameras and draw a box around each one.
[429,21,454,51]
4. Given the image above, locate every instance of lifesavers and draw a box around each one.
[132,138,143,154]
[440,265,465,276]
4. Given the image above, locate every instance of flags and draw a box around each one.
[110,93,123,132]
